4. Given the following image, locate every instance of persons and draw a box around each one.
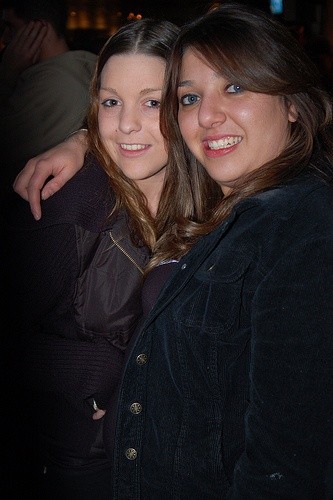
[0,0,100,200]
[0,18,184,500]
[12,4,333,500]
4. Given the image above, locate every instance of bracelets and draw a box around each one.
[64,129,89,142]
